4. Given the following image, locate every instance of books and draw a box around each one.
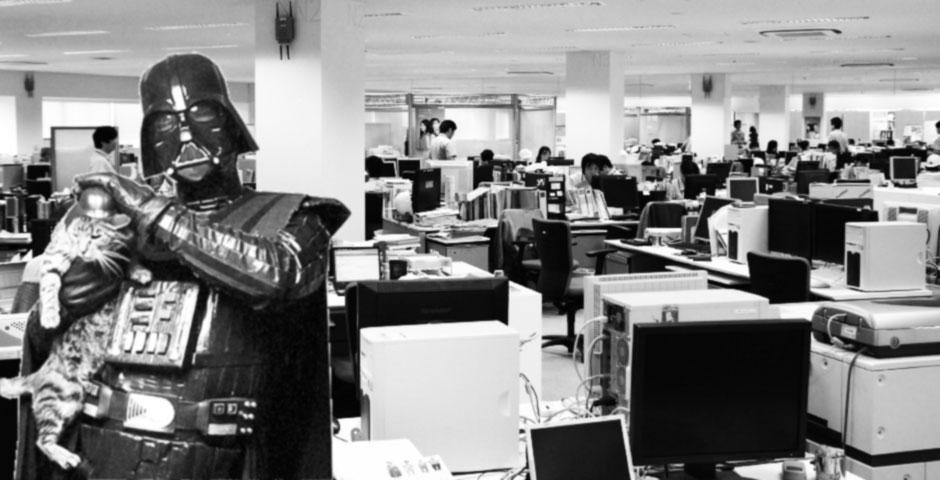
[369,228,422,263]
[400,251,452,272]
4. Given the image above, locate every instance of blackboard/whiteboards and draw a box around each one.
[51,126,119,191]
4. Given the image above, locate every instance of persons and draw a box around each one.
[416,116,496,190]
[642,115,852,172]
[929,122,938,150]
[20,49,353,480]
[81,124,122,178]
[535,146,623,187]
